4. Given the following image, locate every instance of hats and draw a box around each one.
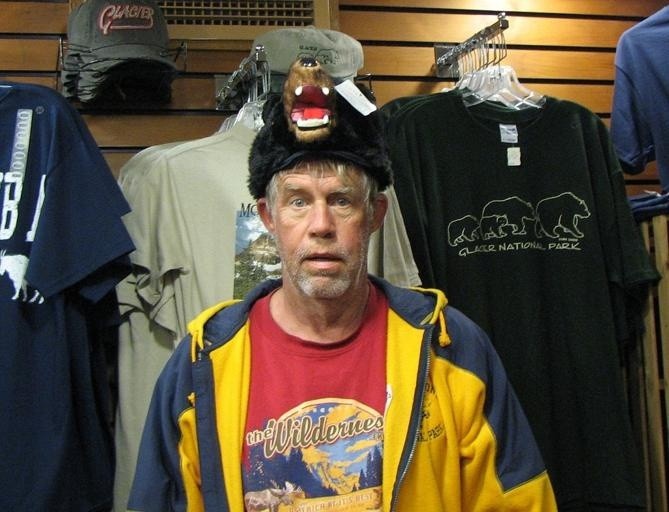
[249,56,394,199]
[251,25,363,94]
[61,0,177,105]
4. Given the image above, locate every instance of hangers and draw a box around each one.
[433,19,543,117]
[213,48,277,132]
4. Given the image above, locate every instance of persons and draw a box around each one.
[123,54,563,511]
